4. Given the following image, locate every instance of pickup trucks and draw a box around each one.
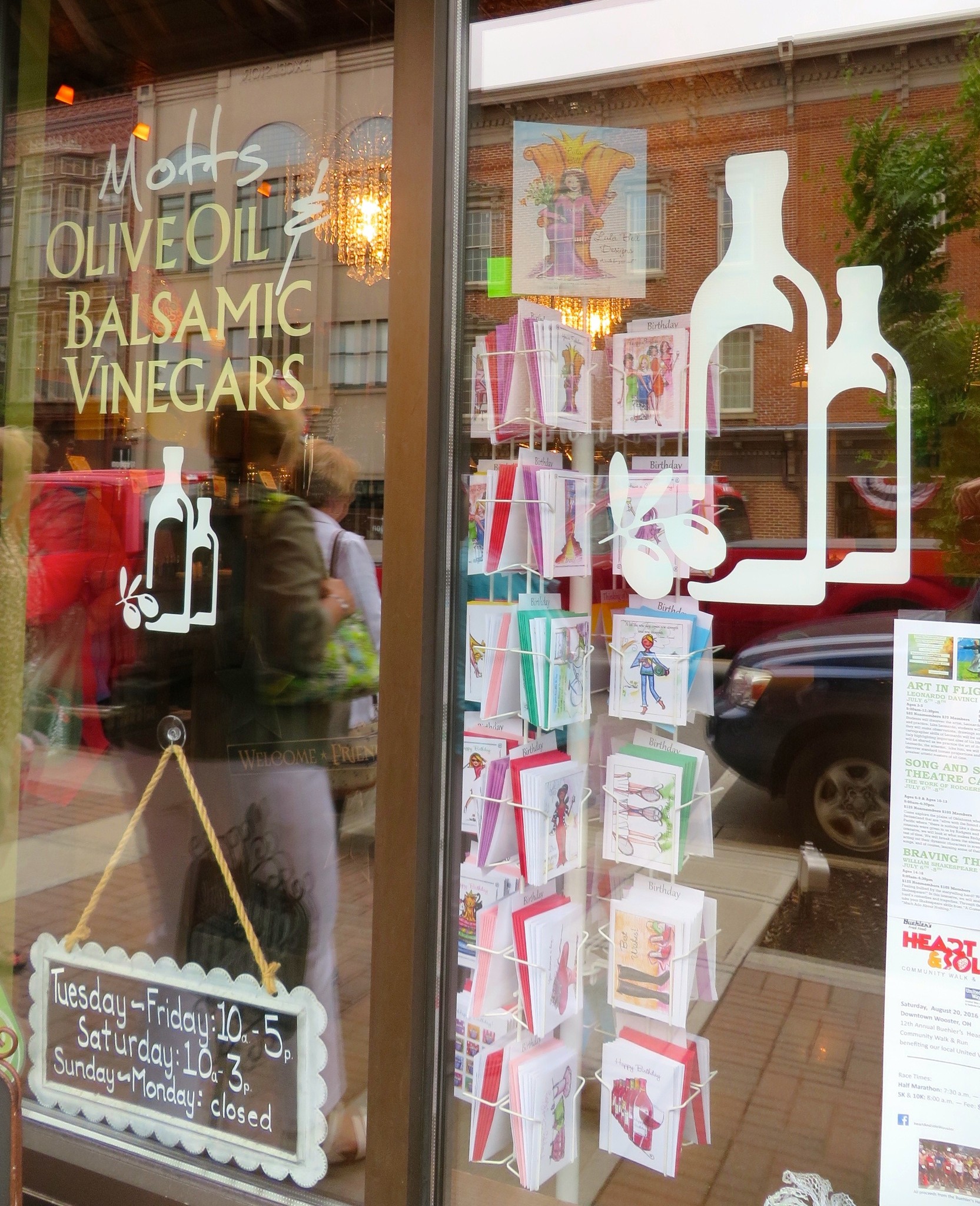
[551,487,965,652]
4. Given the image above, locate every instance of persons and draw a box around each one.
[1,379,134,807]
[0,424,51,978]
[88,369,372,1168]
[276,437,384,853]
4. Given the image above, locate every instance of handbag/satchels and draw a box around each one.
[330,693,377,734]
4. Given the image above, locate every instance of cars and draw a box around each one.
[702,578,980,859]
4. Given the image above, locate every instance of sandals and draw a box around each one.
[321,1107,367,1167]
